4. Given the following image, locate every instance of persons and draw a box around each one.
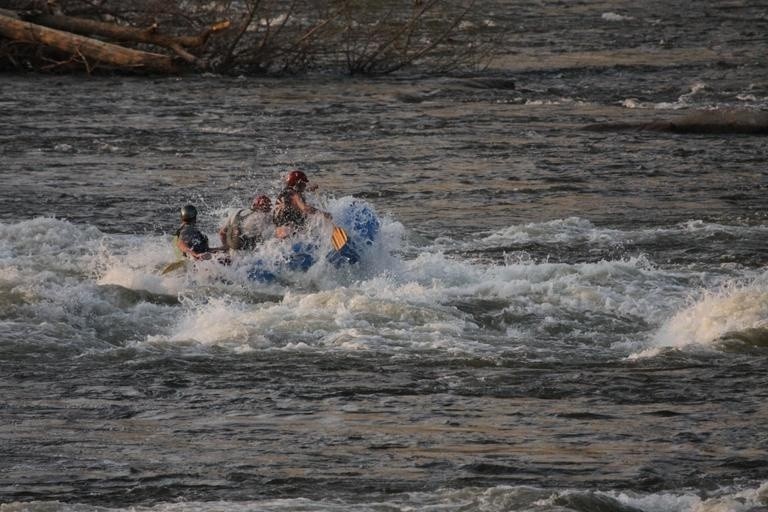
[217,194,276,267]
[271,171,334,237]
[170,202,212,273]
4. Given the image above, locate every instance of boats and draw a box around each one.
[128,201,382,288]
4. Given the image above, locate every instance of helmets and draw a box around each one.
[287,170,309,185]
[181,205,197,219]
[253,196,273,212]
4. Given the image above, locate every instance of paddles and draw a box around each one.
[162,253,211,275]
[329,220,348,251]
